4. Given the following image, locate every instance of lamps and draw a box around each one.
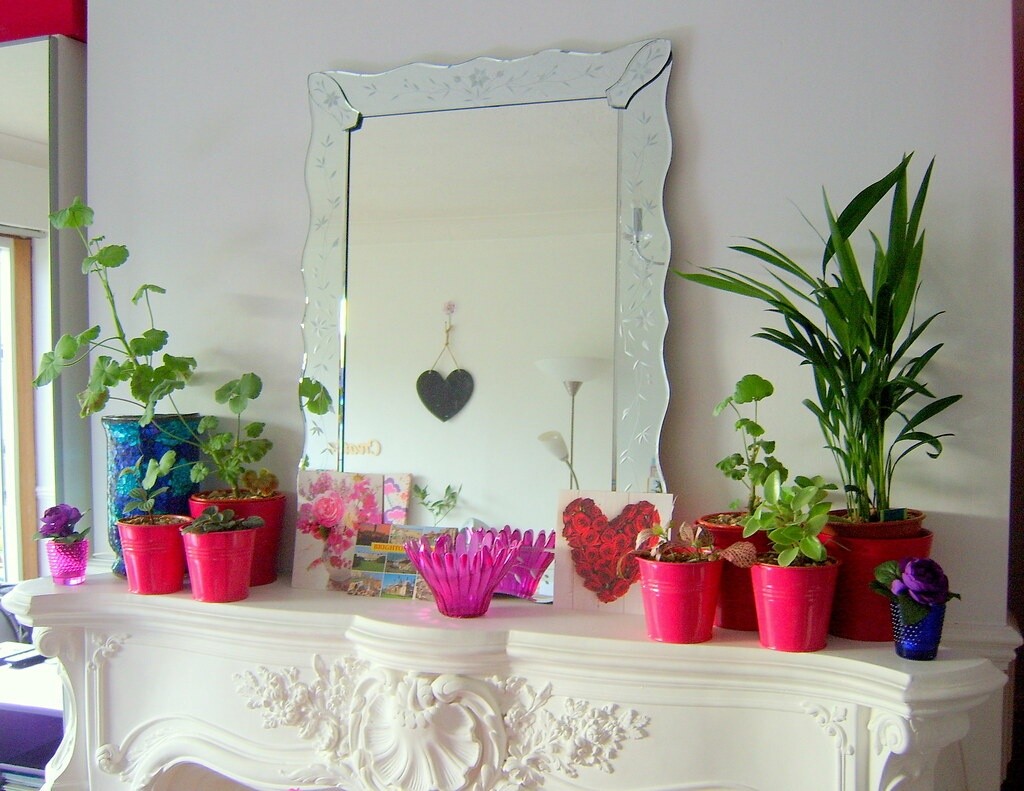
[536,351,591,490]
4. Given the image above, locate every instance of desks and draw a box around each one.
[0,559,1011,791]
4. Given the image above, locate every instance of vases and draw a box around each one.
[47,540,88,587]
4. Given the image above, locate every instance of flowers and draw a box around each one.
[890,601,946,661]
[33,504,92,546]
[870,554,962,625]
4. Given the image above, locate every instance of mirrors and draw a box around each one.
[297,38,673,606]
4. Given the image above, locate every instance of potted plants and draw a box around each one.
[614,516,761,645]
[32,198,287,605]
[741,472,840,654]
[668,146,964,643]
